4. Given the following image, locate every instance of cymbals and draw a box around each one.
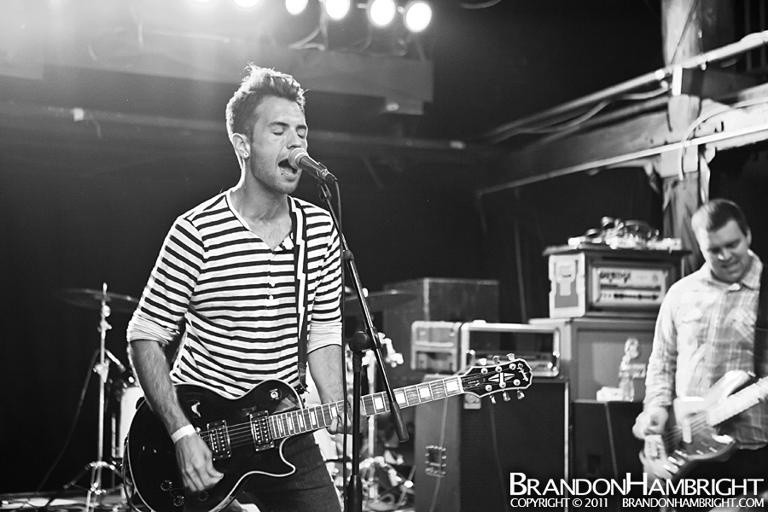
[57,289,138,314]
[342,292,416,316]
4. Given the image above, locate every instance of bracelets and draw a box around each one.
[171,423,196,443]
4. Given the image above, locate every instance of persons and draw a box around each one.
[124,62,367,511]
[627,198,767,510]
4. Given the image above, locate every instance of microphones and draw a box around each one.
[105,349,126,373]
[289,148,338,184]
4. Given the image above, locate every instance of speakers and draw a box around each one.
[413,376,570,512]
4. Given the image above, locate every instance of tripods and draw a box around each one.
[41,303,133,512]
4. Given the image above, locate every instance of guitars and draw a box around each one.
[639,369,767,488]
[123,353,533,512]
